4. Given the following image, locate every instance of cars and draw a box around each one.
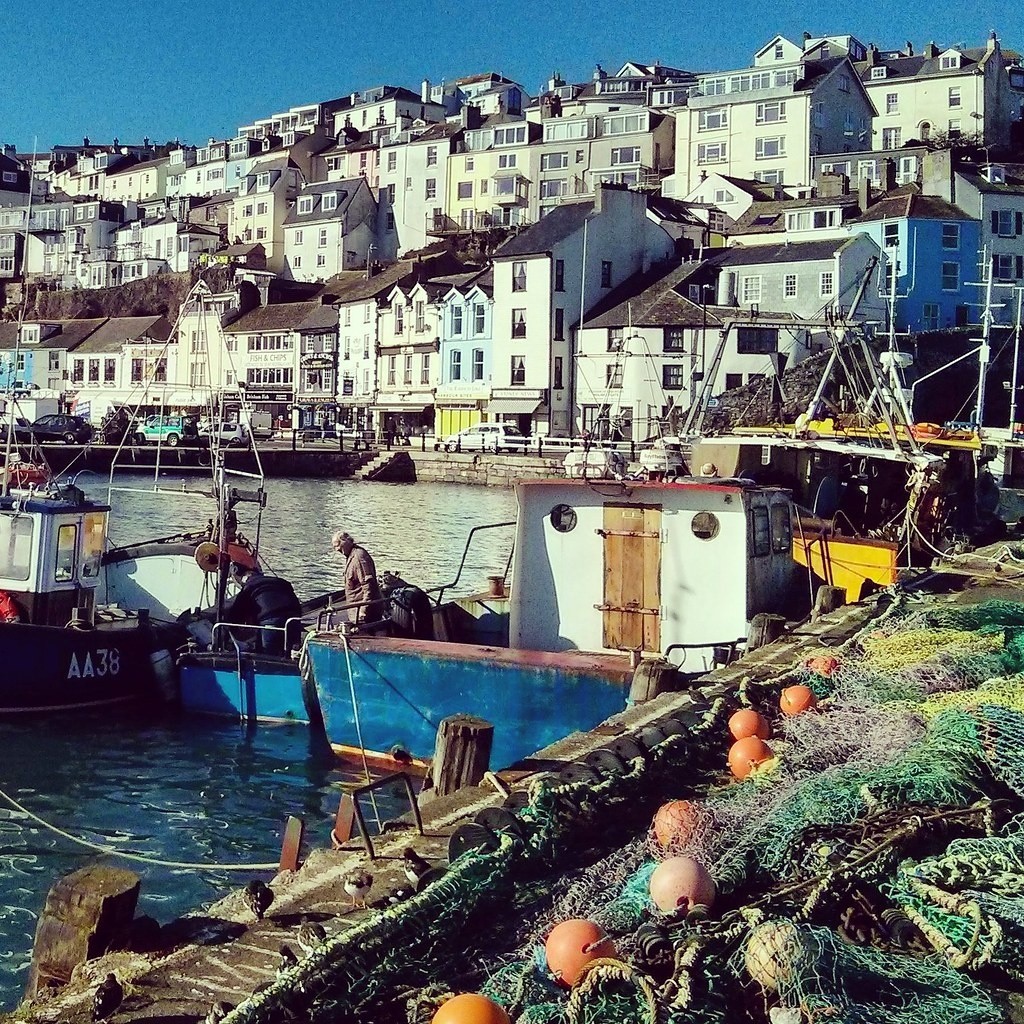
[196,421,250,449]
[444,422,526,452]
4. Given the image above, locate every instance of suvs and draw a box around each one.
[17,413,93,446]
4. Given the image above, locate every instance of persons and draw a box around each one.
[386,417,396,445]
[576,429,591,452]
[228,569,301,654]
[332,532,385,624]
[321,416,331,442]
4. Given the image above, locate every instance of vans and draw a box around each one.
[133,413,201,448]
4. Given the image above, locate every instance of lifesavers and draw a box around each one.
[915,427,947,439]
[195,450,212,467]
[7,468,49,489]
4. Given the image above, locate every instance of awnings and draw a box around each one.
[488,400,543,413]
[368,403,431,412]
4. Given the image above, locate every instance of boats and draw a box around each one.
[0,209,1024,784]
